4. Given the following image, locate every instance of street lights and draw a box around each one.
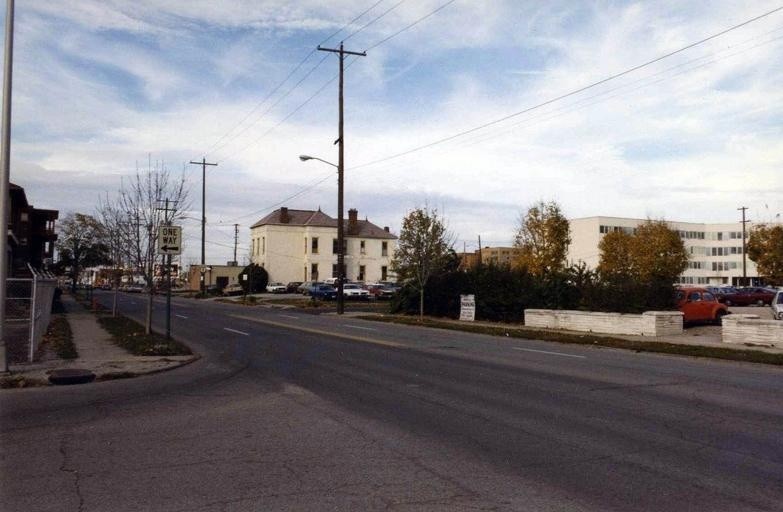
[179,217,205,290]
[300,156,344,314]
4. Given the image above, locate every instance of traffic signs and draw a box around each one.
[158,226,181,255]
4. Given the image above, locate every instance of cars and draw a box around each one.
[674,287,783,326]
[287,277,402,299]
[102,284,110,290]
[222,283,243,296]
[118,284,143,293]
[266,283,288,293]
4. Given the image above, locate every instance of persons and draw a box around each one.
[171,279,176,290]
[156,278,160,289]
